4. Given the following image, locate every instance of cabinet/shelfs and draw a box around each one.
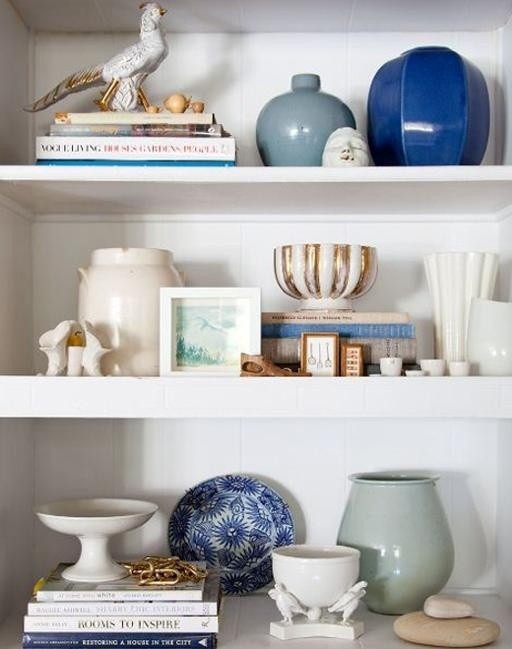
[0,1,512,649]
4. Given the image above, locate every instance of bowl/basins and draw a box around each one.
[273,243,379,312]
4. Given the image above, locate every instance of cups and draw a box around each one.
[420,359,446,376]
[379,358,402,376]
[447,361,471,376]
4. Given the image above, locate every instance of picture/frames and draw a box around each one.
[158,286,262,376]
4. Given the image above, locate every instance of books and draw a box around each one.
[19,560,225,647]
[34,110,239,167]
[261,309,420,375]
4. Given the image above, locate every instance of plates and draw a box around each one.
[167,474,296,597]
